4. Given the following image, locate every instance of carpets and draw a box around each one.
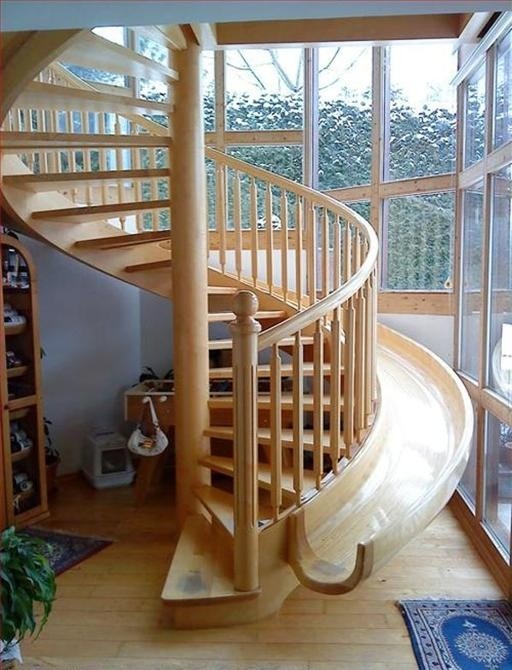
[17,528,113,578]
[397,599,512,670]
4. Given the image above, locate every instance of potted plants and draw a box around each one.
[43,417,62,494]
[1,523,57,670]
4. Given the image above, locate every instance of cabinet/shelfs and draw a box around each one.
[0,232,51,532]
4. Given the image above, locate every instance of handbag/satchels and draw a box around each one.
[127,426,169,456]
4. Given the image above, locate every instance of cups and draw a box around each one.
[11,430,28,443]
[11,439,32,453]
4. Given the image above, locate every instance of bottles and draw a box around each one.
[3,261,30,290]
[13,472,34,494]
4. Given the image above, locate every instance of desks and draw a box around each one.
[122,379,299,498]
